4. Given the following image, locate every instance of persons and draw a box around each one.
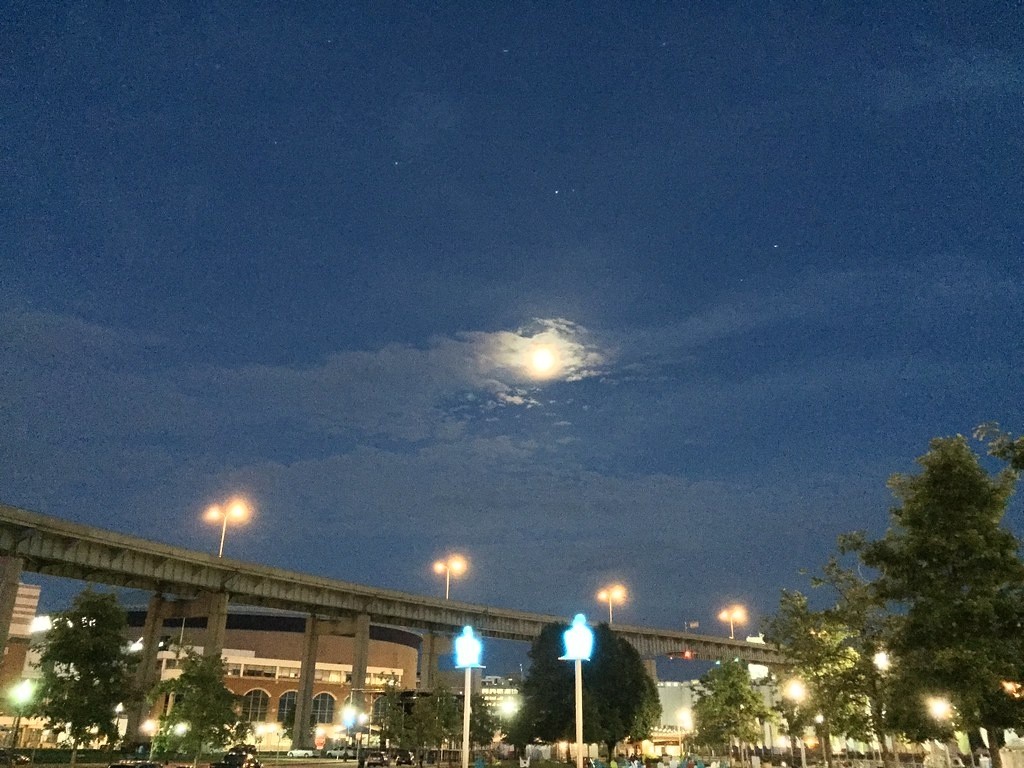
[687,757,694,768]
[419,750,424,768]
[583,746,646,768]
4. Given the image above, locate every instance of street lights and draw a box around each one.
[451,622,488,768]
[596,582,629,621]
[558,610,595,768]
[925,696,956,768]
[713,604,750,638]
[782,677,811,768]
[198,498,256,557]
[429,551,469,601]
